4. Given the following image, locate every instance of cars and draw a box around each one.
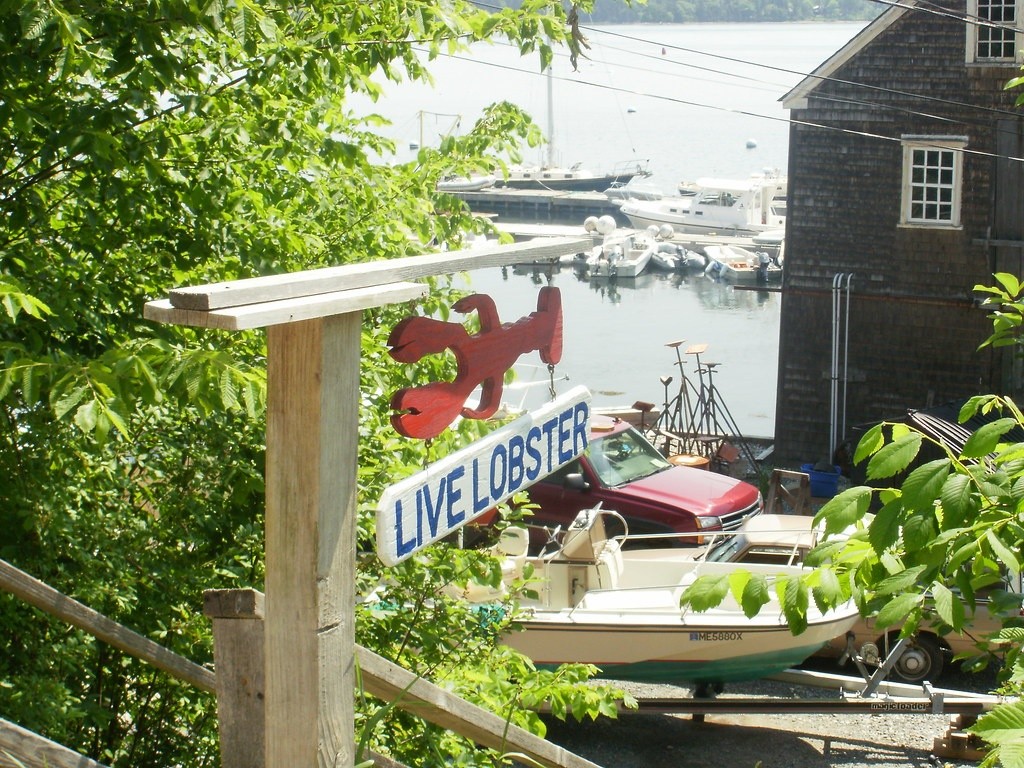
[440,415,765,550]
[617,514,1023,685]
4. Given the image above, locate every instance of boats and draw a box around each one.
[619,168,789,236]
[702,244,781,282]
[367,498,878,685]
[495,159,649,192]
[435,163,493,191]
[653,241,706,271]
[585,231,655,277]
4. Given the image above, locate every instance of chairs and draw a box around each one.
[425,528,530,606]
